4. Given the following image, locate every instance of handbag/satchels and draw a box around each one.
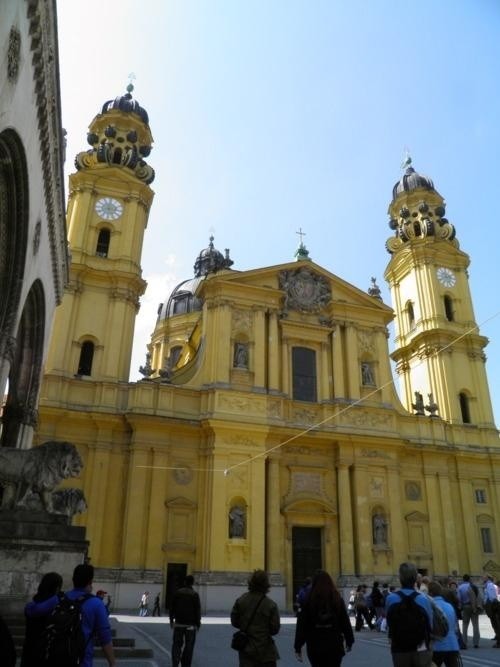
[433,604,448,638]
[231,631,248,652]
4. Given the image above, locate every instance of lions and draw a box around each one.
[0,441,83,516]
[17,488,88,526]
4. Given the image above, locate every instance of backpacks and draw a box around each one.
[386,591,430,650]
[42,592,96,667]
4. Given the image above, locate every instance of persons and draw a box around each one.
[1,563,116,666]
[139,591,150,616]
[168,575,201,667]
[293,561,500,667]
[152,592,160,616]
[230,570,281,666]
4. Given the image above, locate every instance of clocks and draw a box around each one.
[94,194,124,221]
[437,266,457,289]
